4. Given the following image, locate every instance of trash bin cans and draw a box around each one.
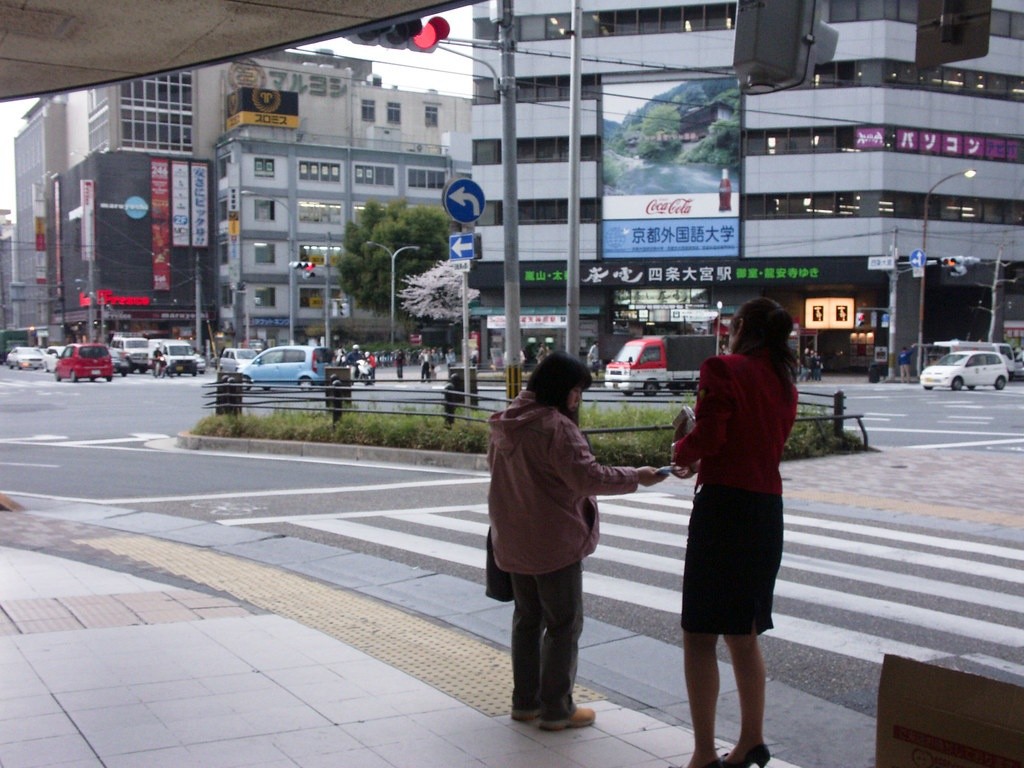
[868,363,879,382]
[449,365,478,410]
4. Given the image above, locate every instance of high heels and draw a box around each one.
[668,743,770,768]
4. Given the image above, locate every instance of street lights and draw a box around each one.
[367,240,421,347]
[915,169,979,380]
[241,190,298,346]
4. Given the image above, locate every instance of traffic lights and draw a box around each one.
[292,260,317,280]
[855,313,867,329]
[343,14,451,54]
[939,255,964,268]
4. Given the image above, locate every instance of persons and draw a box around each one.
[395,348,405,378]
[335,345,378,386]
[536,342,545,364]
[897,344,916,383]
[468,345,480,368]
[799,348,823,382]
[669,299,798,768]
[484,351,670,732]
[416,347,438,384]
[587,341,600,379]
[152,344,167,371]
[445,347,456,379]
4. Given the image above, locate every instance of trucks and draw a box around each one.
[604,334,717,397]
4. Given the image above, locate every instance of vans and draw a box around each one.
[148,338,207,375]
[217,347,263,383]
[110,336,150,375]
[933,338,1024,382]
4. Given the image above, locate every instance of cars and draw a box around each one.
[6,346,47,370]
[55,342,113,383]
[920,350,1009,392]
[235,346,336,394]
[45,345,67,373]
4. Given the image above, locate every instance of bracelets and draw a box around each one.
[689,465,697,474]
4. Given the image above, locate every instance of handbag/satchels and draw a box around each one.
[485,526,516,601]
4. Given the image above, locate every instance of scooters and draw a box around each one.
[152,355,169,378]
[118,358,132,377]
[353,358,374,383]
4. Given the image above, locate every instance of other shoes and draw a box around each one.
[538,707,595,730]
[511,707,543,720]
